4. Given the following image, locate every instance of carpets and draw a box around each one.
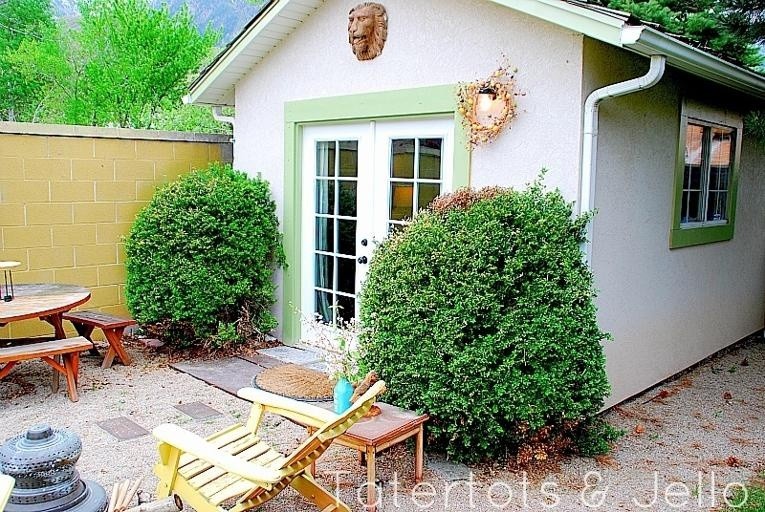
[253,360,337,402]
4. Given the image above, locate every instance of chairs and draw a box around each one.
[152,376,386,512]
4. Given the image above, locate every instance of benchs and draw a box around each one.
[64,311,137,369]
[1,335,94,403]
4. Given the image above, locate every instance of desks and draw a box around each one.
[1,279,90,393]
[305,400,430,512]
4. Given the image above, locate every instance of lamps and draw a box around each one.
[477,83,499,112]
